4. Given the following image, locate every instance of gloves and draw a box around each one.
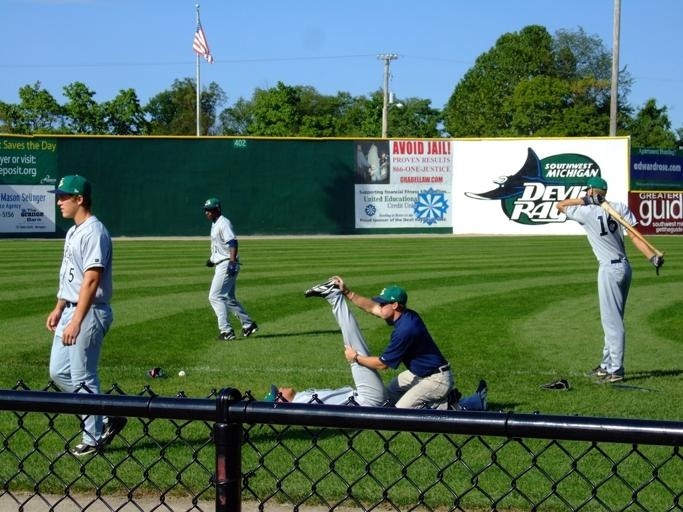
[227,262,236,275]
[583,195,605,205]
[649,255,665,268]
[206,259,215,267]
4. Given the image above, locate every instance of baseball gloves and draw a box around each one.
[650,256,665,277]
[581,195,603,205]
[226,260,242,277]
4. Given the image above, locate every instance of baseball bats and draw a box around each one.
[598,200,665,258]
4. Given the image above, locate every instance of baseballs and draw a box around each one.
[179,371,185,377]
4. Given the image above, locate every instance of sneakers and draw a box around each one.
[586,367,608,377]
[66,443,97,455]
[97,416,127,446]
[305,278,341,298]
[217,330,235,342]
[475,379,487,410]
[448,388,463,411]
[592,371,624,384]
[238,323,258,337]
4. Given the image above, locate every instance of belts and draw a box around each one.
[428,364,450,374]
[64,301,80,308]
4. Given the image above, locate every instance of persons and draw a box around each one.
[265,278,389,408]
[45,175,127,455]
[204,198,258,340]
[556,179,664,383]
[327,274,488,412]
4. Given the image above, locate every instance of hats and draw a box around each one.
[582,177,607,191]
[264,384,278,401]
[203,199,221,210]
[371,284,408,304]
[49,176,92,198]
